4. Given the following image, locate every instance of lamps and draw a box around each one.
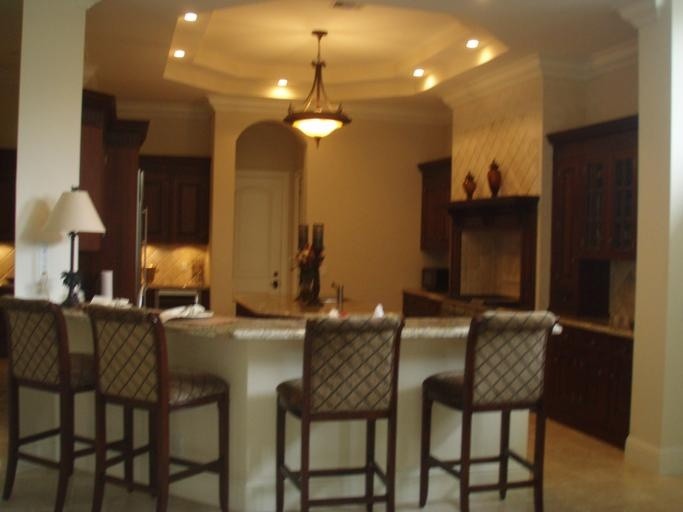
[37,186,107,310]
[281,29,352,151]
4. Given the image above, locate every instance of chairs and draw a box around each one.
[85,305,229,512]
[1,297,154,511]
[273,314,406,511]
[419,311,558,511]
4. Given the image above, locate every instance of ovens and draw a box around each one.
[153,288,201,311]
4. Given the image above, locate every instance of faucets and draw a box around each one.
[330,279,344,312]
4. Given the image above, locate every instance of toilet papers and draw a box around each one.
[99,269,114,300]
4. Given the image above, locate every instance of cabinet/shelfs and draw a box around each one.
[138,164,208,246]
[570,145,637,259]
